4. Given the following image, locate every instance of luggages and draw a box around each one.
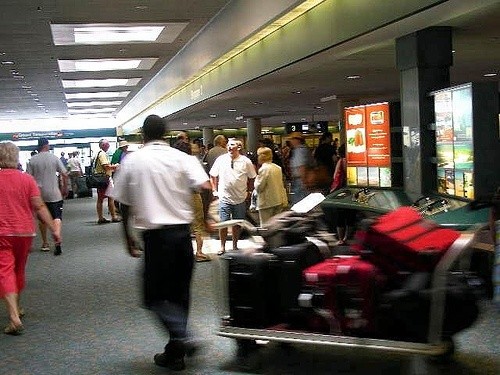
[267,242,323,323]
[298,255,386,334]
[361,206,460,271]
[227,253,279,327]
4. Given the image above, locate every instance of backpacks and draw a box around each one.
[258,211,318,247]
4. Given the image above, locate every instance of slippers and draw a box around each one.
[2,325,24,335]
[196,255,213,262]
[217,250,225,255]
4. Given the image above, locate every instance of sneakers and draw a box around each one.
[40,242,50,251]
[112,219,121,222]
[54,242,62,256]
[97,218,110,224]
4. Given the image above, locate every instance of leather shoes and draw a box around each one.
[154,353,185,371]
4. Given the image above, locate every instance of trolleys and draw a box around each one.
[210,219,496,371]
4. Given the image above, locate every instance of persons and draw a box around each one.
[26,138,68,255]
[60,152,67,168]
[205,135,228,171]
[0,142,62,334]
[330,143,349,246]
[176,132,189,144]
[175,142,212,263]
[209,139,257,255]
[254,146,288,251]
[287,131,314,207]
[488,186,500,305]
[193,139,200,147]
[93,139,123,224]
[313,132,339,198]
[70,151,85,198]
[104,114,213,371]
[110,141,129,220]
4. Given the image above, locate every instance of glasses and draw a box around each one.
[231,160,233,169]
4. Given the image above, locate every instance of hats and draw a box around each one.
[119,141,129,148]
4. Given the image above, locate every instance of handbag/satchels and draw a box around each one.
[86,151,110,189]
[379,269,480,344]
